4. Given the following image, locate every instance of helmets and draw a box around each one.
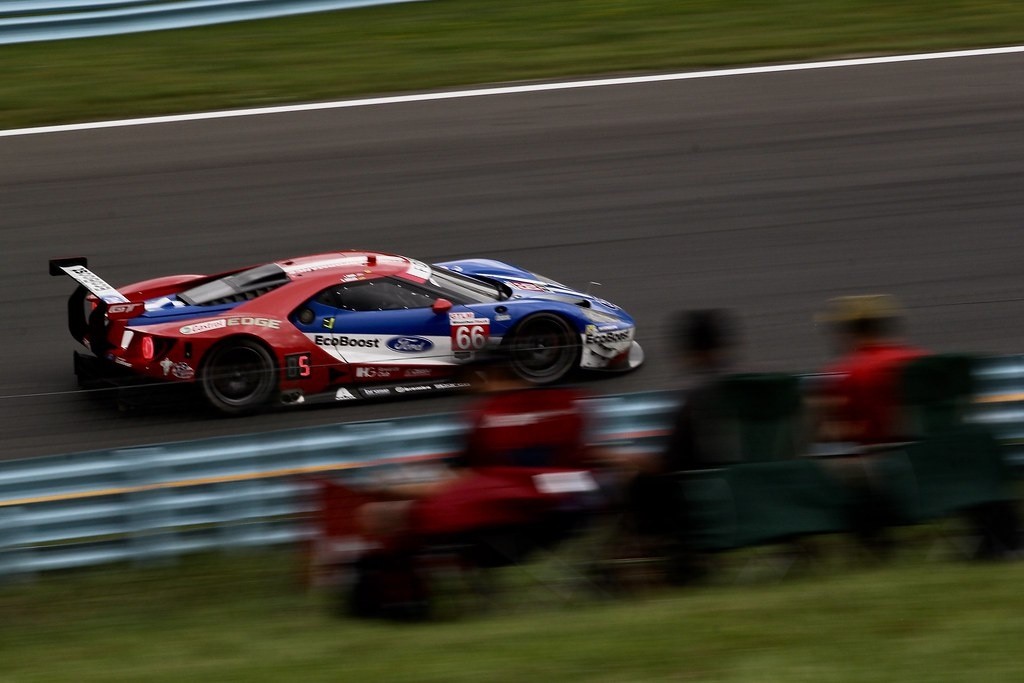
[811,294,906,323]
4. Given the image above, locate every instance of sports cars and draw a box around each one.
[48,247,647,418]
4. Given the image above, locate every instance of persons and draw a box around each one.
[373,297,990,609]
